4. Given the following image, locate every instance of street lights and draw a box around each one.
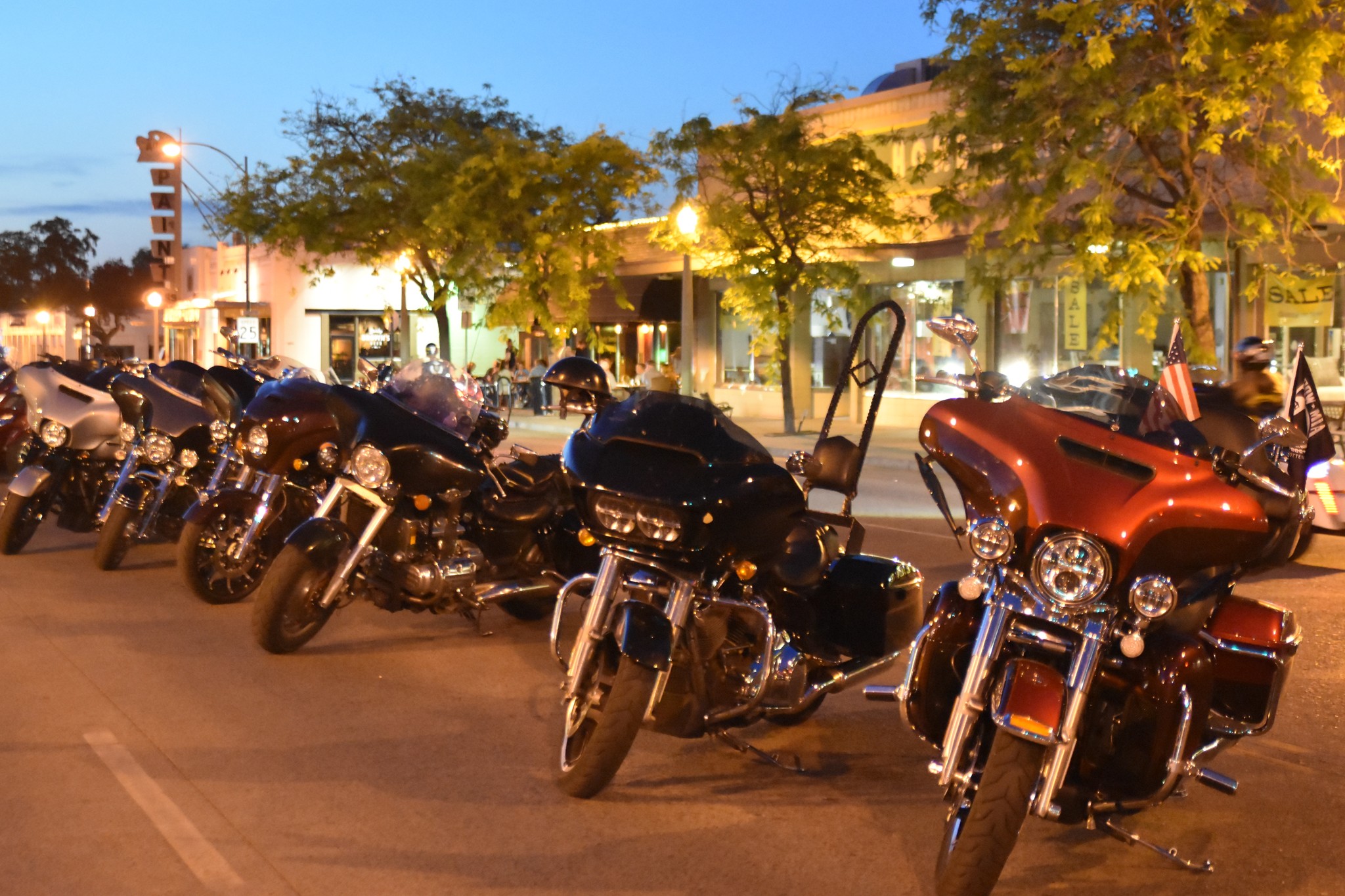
[160,139,252,359]
[148,291,162,362]
[35,310,50,355]
[677,202,702,396]
[393,251,414,367]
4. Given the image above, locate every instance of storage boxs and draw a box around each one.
[1208,590,1304,722]
[821,548,925,662]
[474,403,511,447]
[546,504,604,590]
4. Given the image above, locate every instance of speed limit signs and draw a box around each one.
[235,317,259,345]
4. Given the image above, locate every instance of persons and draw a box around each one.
[1228,337,1277,399]
[634,360,679,395]
[425,344,440,362]
[461,339,555,417]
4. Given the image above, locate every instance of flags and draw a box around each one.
[1138,325,1202,436]
[1285,348,1337,473]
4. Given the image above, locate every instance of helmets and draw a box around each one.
[1233,336,1270,362]
[544,355,611,392]
[425,343,437,355]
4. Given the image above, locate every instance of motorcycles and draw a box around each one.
[537,357,922,797]
[249,355,585,654]
[860,316,1305,896]
[0,336,359,601]
